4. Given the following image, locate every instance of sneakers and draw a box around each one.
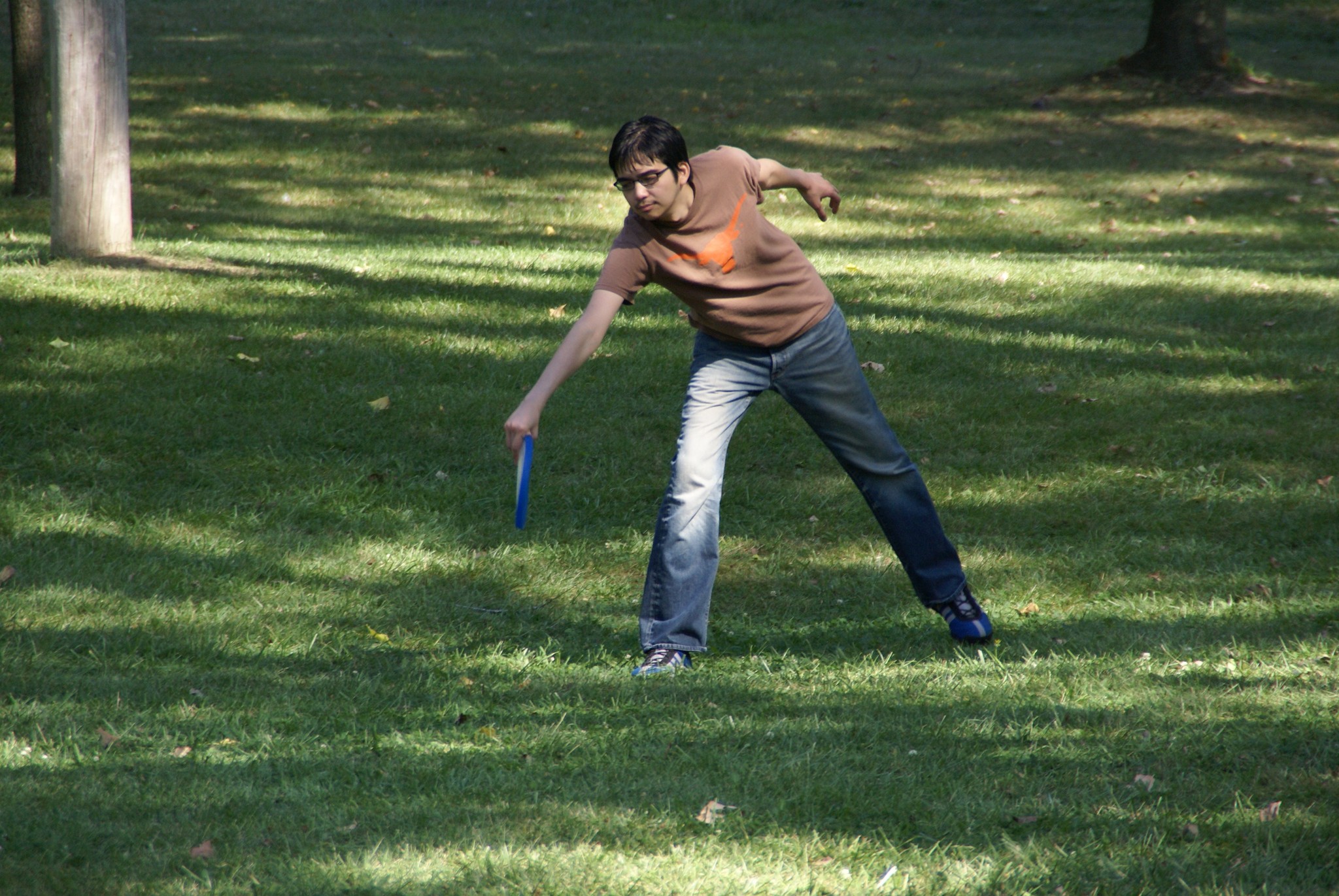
[631,648,694,681]
[931,585,992,644]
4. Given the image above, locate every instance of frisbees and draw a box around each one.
[515,434,534,529]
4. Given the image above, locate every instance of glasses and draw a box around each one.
[614,167,669,191]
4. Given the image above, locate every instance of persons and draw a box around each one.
[503,116,993,680]
